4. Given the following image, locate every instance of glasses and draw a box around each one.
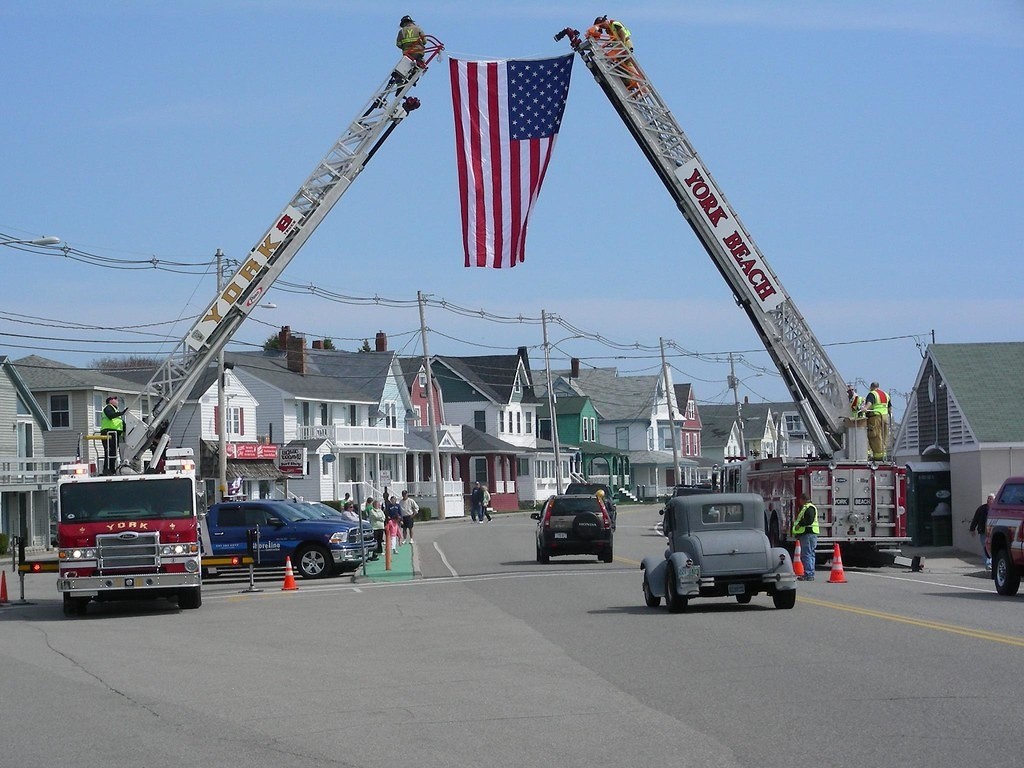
[848,389,854,392]
[350,506,354,509]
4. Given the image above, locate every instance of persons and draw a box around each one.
[341,493,350,509]
[369,501,386,556]
[388,496,402,522]
[863,382,892,461]
[399,490,419,544]
[343,502,359,518]
[471,481,493,524]
[395,15,427,96]
[364,497,374,514]
[970,493,995,570]
[792,493,820,581]
[389,513,403,554]
[100,396,128,473]
[847,384,865,418]
[595,15,635,89]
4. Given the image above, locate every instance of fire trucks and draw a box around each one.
[554,20,913,566]
[17,34,448,619]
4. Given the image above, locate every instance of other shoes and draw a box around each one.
[478,521,484,524]
[402,541,408,544]
[986,563,992,570]
[869,457,882,461]
[377,553,385,556]
[797,573,814,581]
[394,551,399,554]
[487,519,492,523]
[409,540,414,544]
[471,521,477,524]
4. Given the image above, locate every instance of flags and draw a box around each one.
[448,53,575,268]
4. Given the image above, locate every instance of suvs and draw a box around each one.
[983,476,1024,597]
[560,481,621,532]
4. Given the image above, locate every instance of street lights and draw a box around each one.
[219,295,278,501]
[544,334,587,495]
[733,373,765,462]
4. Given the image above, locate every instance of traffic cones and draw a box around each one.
[280,555,300,591]
[0,571,13,606]
[826,543,849,583]
[792,540,805,578]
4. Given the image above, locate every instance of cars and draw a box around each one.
[639,492,799,613]
[530,493,616,564]
[198,499,380,579]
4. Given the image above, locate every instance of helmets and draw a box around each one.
[596,490,605,498]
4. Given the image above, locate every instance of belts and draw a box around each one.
[868,415,876,418]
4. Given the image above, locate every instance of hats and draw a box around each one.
[402,490,408,495]
[105,396,117,405]
[801,493,811,502]
